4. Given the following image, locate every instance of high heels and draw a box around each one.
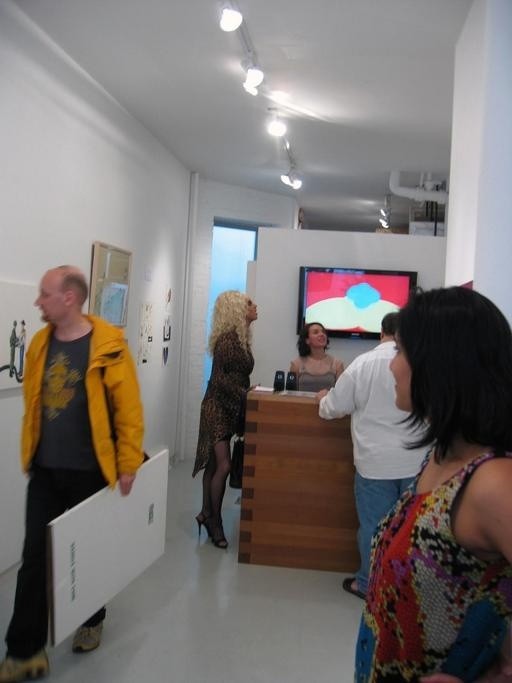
[196,512,229,548]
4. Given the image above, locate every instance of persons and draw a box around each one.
[289,322,344,393]
[316,310,437,598]
[192,288,259,548]
[16,319,27,377]
[0,264,146,682]
[9,320,18,377]
[352,285,512,683]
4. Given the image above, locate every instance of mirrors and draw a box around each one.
[88,240,133,328]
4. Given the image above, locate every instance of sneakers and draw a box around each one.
[0,650,49,682]
[71,620,103,653]
[343,577,365,598]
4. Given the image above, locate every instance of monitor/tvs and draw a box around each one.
[297,266,417,340]
